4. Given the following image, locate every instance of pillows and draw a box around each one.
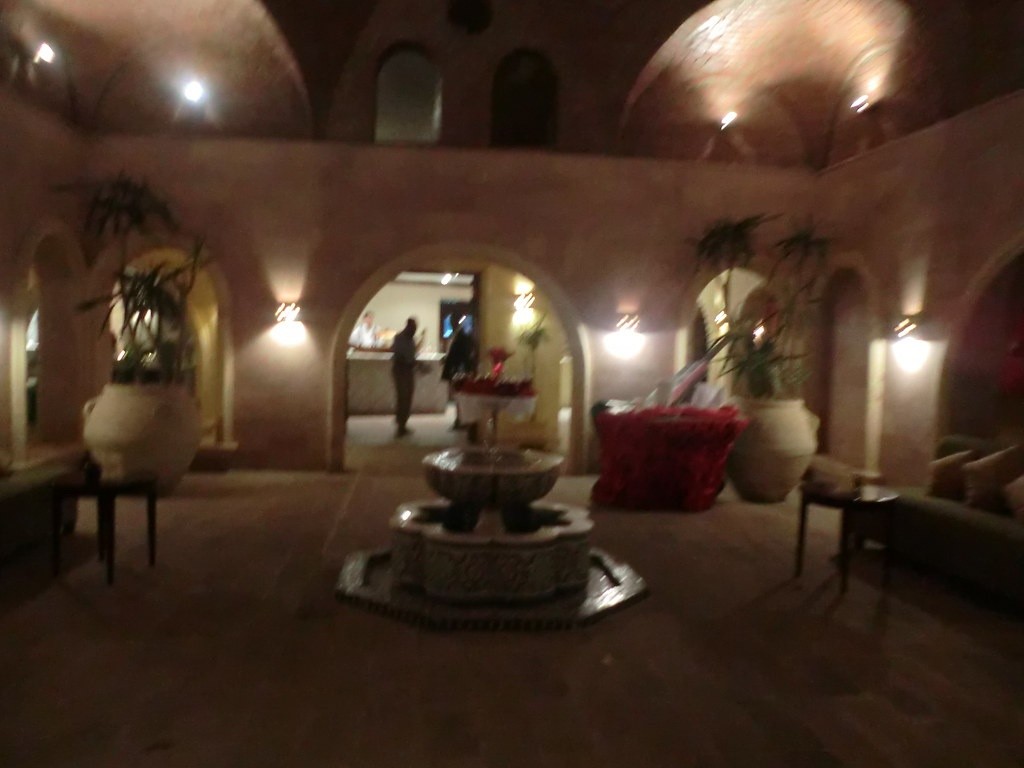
[1001,473,1024,524]
[923,447,983,503]
[958,444,1021,512]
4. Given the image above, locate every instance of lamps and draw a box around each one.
[896,312,921,342]
[616,311,641,335]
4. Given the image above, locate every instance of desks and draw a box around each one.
[45,469,159,586]
[794,481,900,591]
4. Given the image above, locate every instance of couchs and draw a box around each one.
[855,434,1024,618]
[0,451,79,565]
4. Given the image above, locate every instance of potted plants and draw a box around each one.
[687,210,838,504]
[69,171,218,498]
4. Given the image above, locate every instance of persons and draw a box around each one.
[441,312,478,430]
[390,318,425,438]
[349,312,385,352]
